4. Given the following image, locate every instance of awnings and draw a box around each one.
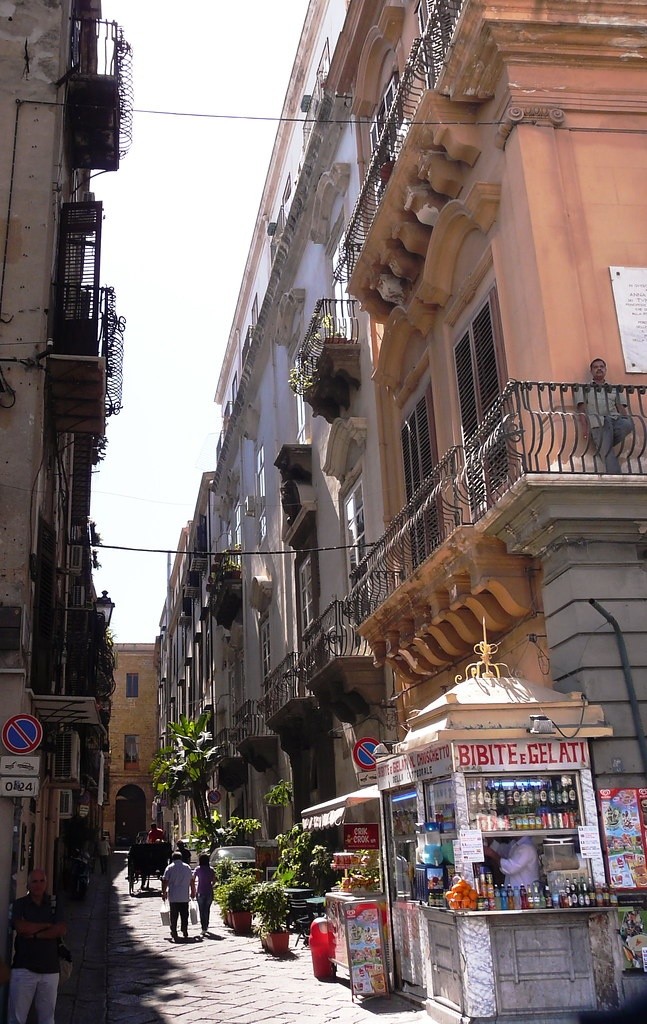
[301,784,380,831]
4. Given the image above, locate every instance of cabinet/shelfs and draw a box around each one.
[469,804,579,837]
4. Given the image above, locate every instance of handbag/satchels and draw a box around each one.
[190,899,198,924]
[160,901,171,926]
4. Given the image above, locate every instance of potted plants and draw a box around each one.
[214,857,264,933]
[223,557,242,579]
[242,882,293,956]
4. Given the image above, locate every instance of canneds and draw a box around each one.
[509,815,542,830]
[479,816,489,831]
[474,871,496,911]
[333,853,356,864]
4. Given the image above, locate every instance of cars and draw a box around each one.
[172,839,209,870]
[209,845,255,869]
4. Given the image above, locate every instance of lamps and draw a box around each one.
[373,740,398,757]
[527,716,556,735]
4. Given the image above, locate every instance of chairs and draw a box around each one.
[289,892,315,947]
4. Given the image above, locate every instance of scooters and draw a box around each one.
[68,849,96,900]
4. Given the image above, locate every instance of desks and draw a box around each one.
[285,889,326,945]
[266,867,278,881]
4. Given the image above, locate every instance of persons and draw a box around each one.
[192,854,218,934]
[148,823,164,842]
[574,358,632,473]
[97,837,111,872]
[484,836,539,889]
[162,852,195,936]
[174,840,191,865]
[7,869,66,1024]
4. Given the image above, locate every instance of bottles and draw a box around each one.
[428,889,449,906]
[392,809,417,836]
[475,867,619,911]
[468,775,582,832]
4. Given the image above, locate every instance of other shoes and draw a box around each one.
[183,932,188,936]
[171,932,178,937]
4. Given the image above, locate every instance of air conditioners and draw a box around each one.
[71,585,85,606]
[52,732,81,782]
[58,790,73,819]
[70,545,82,568]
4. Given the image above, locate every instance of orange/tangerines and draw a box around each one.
[446,879,478,911]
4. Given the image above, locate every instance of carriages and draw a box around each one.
[121,831,174,896]
